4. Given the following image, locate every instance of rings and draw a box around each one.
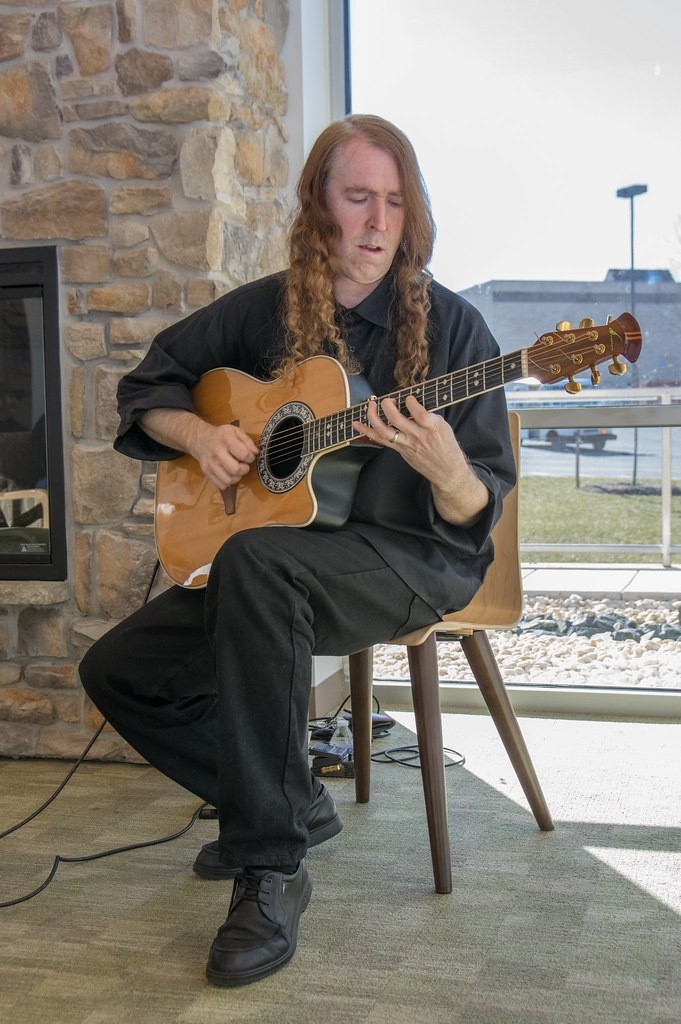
[389,427,399,443]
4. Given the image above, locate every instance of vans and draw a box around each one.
[505,379,618,452]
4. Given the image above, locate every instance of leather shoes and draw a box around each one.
[192,783,344,880]
[205,859,313,986]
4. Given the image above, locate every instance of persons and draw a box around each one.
[77,113,518,987]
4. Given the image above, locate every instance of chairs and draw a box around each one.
[349,409,557,893]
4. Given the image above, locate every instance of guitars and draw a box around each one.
[152,309,643,593]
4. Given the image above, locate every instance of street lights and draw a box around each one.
[616,184,650,388]
